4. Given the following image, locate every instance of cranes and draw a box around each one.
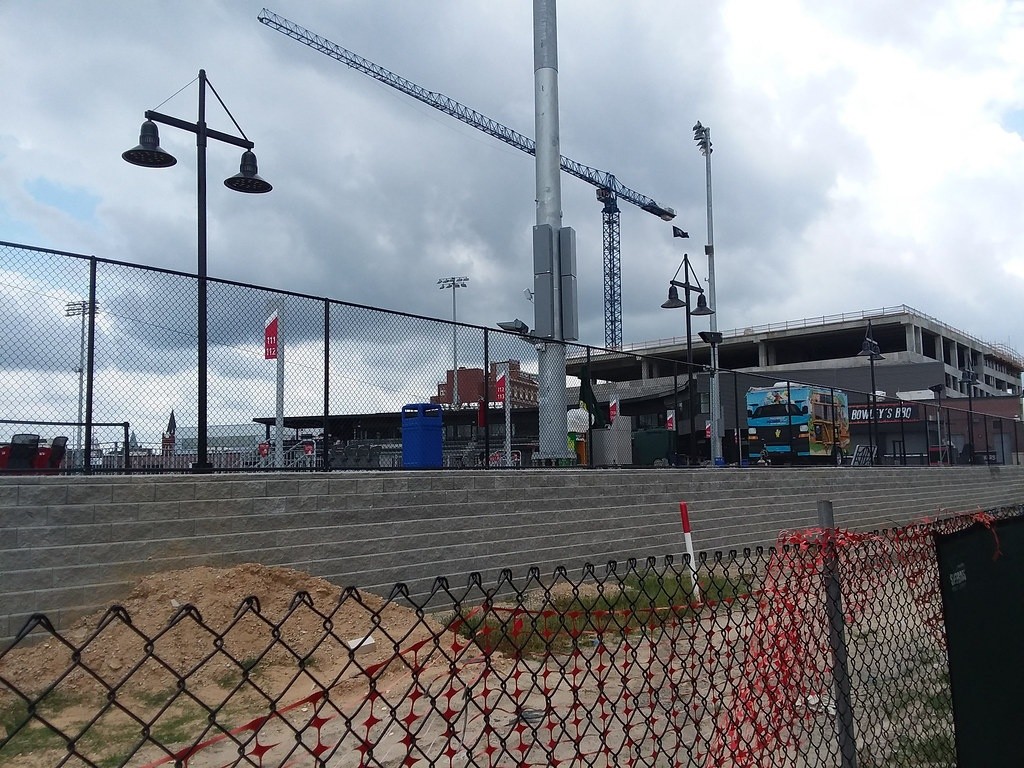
[258,5,677,421]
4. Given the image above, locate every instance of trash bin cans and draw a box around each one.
[402,404,443,470]
[632,428,676,468]
[714,457,724,465]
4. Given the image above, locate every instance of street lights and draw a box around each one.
[122,69,274,472]
[661,253,716,465]
[691,118,724,465]
[64,300,100,467]
[957,362,981,466]
[436,273,470,412]
[857,317,884,465]
